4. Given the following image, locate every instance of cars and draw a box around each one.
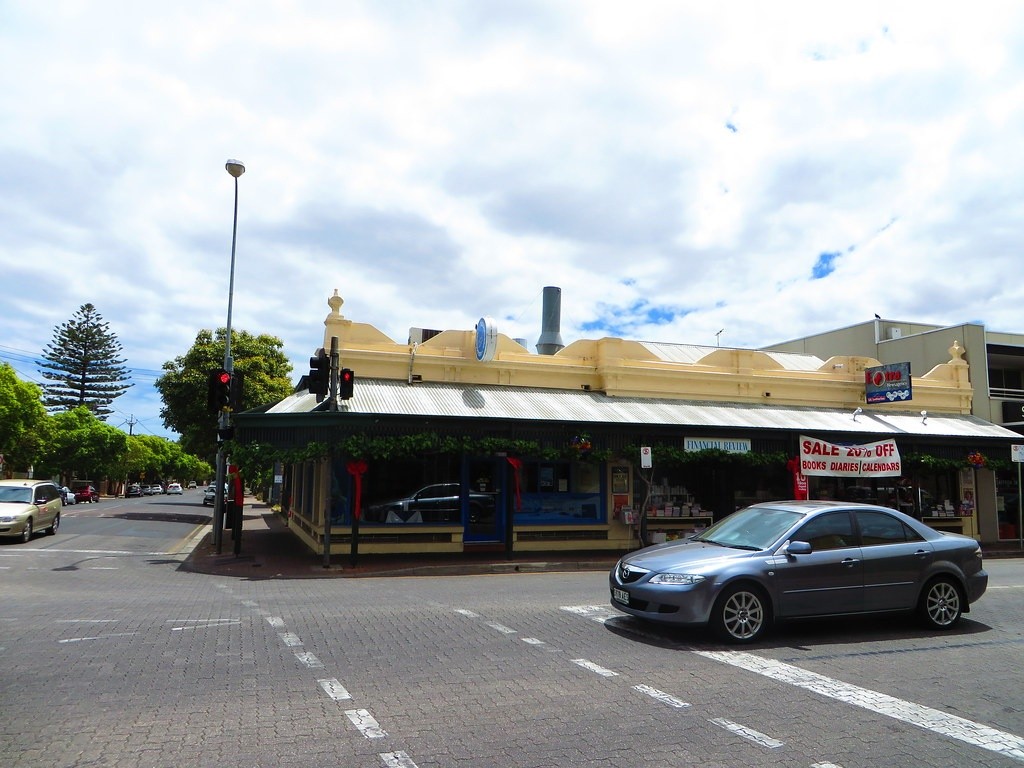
[140,485,153,496]
[203,481,229,506]
[375,482,496,525]
[70,486,100,503]
[124,485,144,498]
[151,484,163,495]
[166,482,184,496]
[55,486,76,506]
[608,500,988,647]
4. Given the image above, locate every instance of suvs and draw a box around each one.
[0,479,63,543]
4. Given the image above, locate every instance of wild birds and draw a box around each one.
[875,313,881,319]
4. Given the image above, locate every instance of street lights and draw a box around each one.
[210,156,245,552]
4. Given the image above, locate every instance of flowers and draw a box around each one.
[966,451,984,471]
[570,433,594,456]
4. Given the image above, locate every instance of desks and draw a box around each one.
[386,508,459,542]
[920,515,974,539]
[642,516,713,547]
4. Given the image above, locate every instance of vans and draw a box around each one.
[189,480,196,489]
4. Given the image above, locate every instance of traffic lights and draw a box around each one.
[340,369,353,397]
[219,369,231,406]
[307,355,322,393]
[207,376,219,413]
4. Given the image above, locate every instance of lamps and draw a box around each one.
[832,364,843,369]
[853,407,863,421]
[920,410,927,423]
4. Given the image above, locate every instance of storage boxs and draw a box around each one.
[647,531,666,543]
